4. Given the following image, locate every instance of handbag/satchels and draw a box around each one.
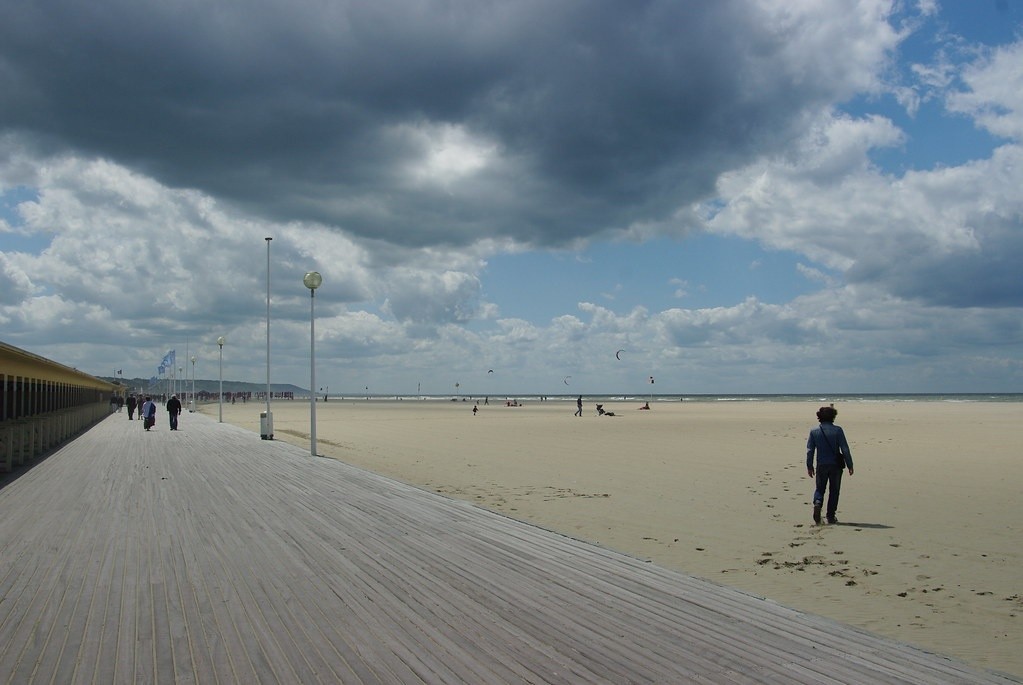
[833,453,846,470]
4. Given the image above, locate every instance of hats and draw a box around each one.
[171,395,177,398]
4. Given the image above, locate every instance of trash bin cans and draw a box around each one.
[259,410,274,440]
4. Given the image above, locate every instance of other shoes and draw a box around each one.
[828,516,837,523]
[814,504,821,522]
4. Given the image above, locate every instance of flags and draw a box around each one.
[147,350,174,384]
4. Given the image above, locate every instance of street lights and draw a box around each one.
[304,269,323,457]
[265,236,273,440]
[190,356,198,413]
[178,367,183,407]
[217,337,225,423]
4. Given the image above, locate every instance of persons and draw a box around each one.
[545,396,547,401]
[645,401,648,407]
[110,393,117,413]
[117,396,124,412]
[806,406,854,523]
[574,395,583,417]
[506,397,507,399]
[540,396,543,401]
[132,391,293,406]
[142,396,156,431]
[507,400,517,406]
[484,397,490,405]
[473,406,478,416]
[230,393,235,405]
[166,394,181,431]
[126,393,137,420]
[477,400,479,405]
[136,394,145,420]
[595,404,605,416]
